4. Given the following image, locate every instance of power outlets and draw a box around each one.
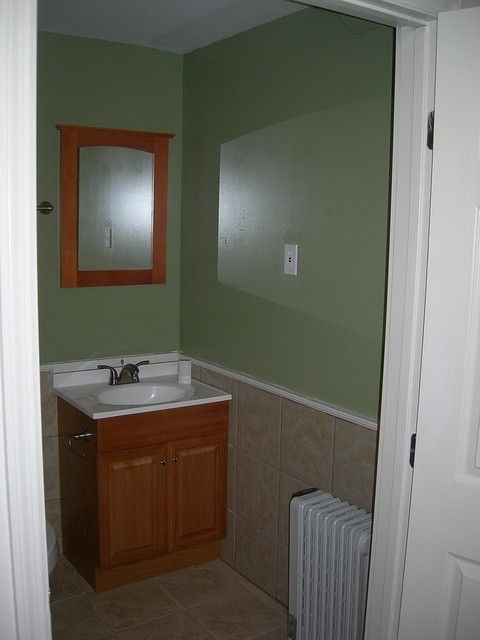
[283,243,298,276]
[104,226,111,248]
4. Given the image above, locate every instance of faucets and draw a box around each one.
[119,363,139,383]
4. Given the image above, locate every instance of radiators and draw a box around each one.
[287,487,373,640]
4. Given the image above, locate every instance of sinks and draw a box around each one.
[97,383,194,405]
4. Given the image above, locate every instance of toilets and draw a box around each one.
[46,520,60,573]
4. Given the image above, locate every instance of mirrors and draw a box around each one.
[55,124,175,289]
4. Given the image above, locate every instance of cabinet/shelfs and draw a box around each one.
[56,394,231,595]
